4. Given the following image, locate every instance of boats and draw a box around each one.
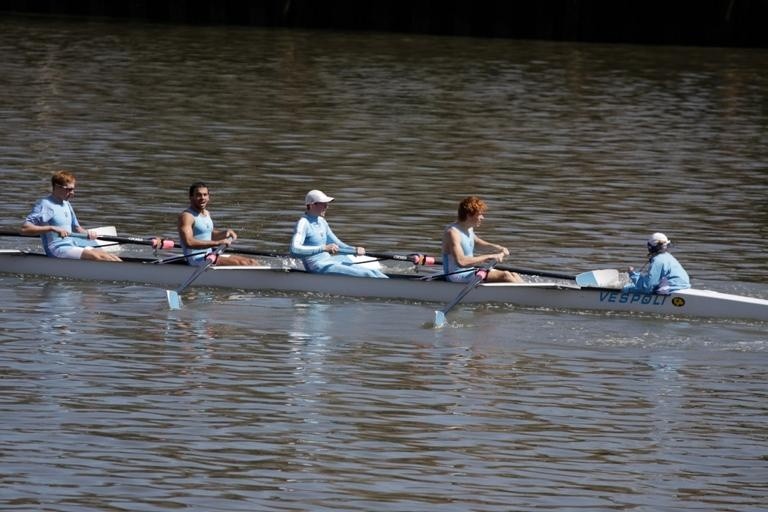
[0,246,768,326]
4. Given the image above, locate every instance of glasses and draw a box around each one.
[55,184,74,192]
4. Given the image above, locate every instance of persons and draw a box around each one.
[148,287,228,406]
[26,282,135,410]
[440,195,525,285]
[405,304,499,433]
[287,189,391,279]
[19,168,125,262]
[626,319,696,498]
[268,295,348,498]
[618,232,691,296]
[176,180,256,267]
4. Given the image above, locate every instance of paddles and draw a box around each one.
[434,252,504,330]
[166,237,233,311]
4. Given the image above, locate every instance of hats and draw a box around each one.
[305,189,336,205]
[648,233,671,248]
